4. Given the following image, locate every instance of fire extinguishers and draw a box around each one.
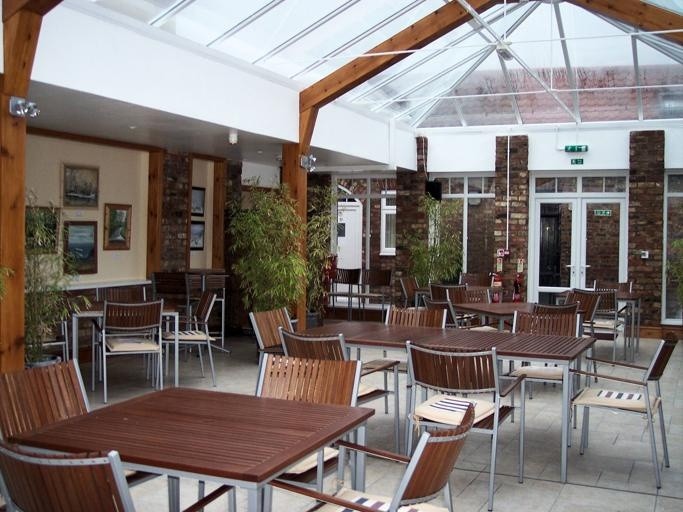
[487,270,504,302]
[511,272,525,302]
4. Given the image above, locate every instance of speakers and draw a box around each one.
[425,180,442,203]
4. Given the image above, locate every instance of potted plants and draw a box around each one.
[223,175,346,365]
[25,186,80,372]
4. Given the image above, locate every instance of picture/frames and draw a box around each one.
[62,221,98,275]
[102,202,132,251]
[60,164,100,211]
[25,205,62,255]
